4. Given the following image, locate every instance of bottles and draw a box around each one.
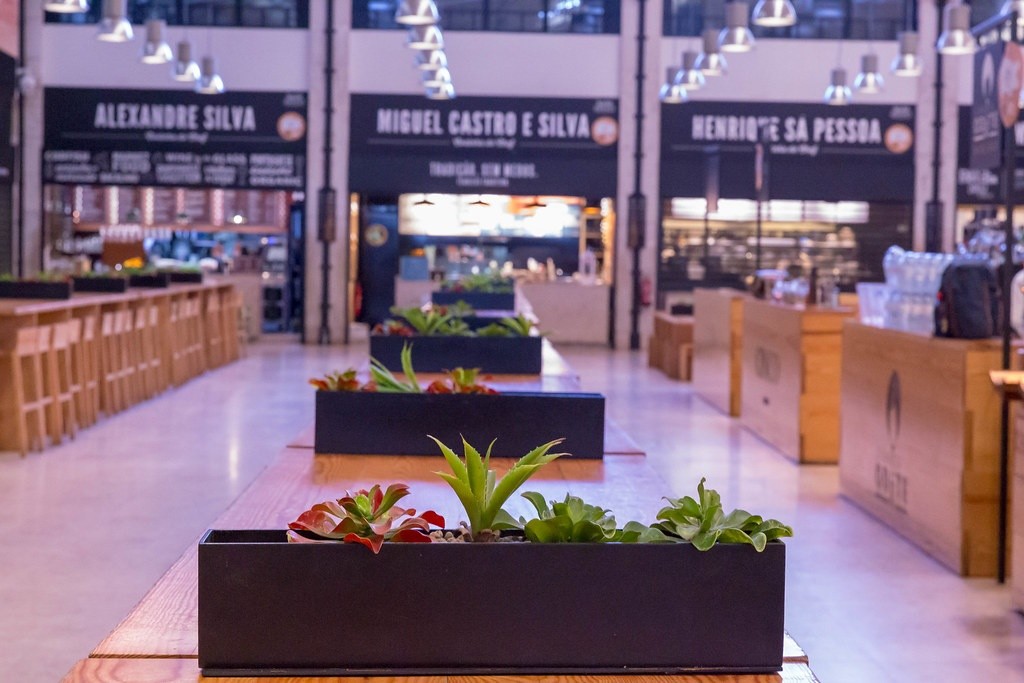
[856,247,953,333]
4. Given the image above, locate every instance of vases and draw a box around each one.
[313,388,606,460]
[432,291,515,311]
[0,280,72,301]
[197,527,788,675]
[128,277,170,289]
[170,274,202,283]
[72,277,130,293]
[370,332,544,374]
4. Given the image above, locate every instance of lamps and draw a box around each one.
[935,0,982,55]
[41,0,90,15]
[413,49,448,69]
[891,30,923,77]
[695,26,729,76]
[675,52,706,90]
[405,25,444,51]
[824,69,855,106]
[716,2,756,53]
[194,0,224,95]
[426,84,456,101]
[658,65,690,105]
[169,40,201,82]
[751,0,797,27]
[394,0,442,26]
[138,19,173,64]
[854,54,886,95]
[421,67,451,87]
[95,0,134,44]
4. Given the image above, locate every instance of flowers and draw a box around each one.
[429,274,516,293]
[306,338,606,393]
[191,430,793,553]
[125,264,169,277]
[368,299,557,337]
[166,264,203,275]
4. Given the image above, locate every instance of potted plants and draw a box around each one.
[0,269,72,282]
[69,269,129,280]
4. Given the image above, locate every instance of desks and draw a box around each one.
[0,281,1024,683]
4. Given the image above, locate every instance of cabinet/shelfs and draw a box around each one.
[581,214,604,277]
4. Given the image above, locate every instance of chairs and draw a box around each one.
[193,298,207,374]
[114,310,131,414]
[121,309,139,409]
[99,310,120,416]
[181,298,200,380]
[220,291,236,363]
[39,324,62,450]
[231,290,250,360]
[52,322,77,446]
[82,314,97,430]
[149,305,164,398]
[65,318,88,434]
[170,300,186,389]
[0,327,47,458]
[134,306,151,400]
[205,292,222,368]
